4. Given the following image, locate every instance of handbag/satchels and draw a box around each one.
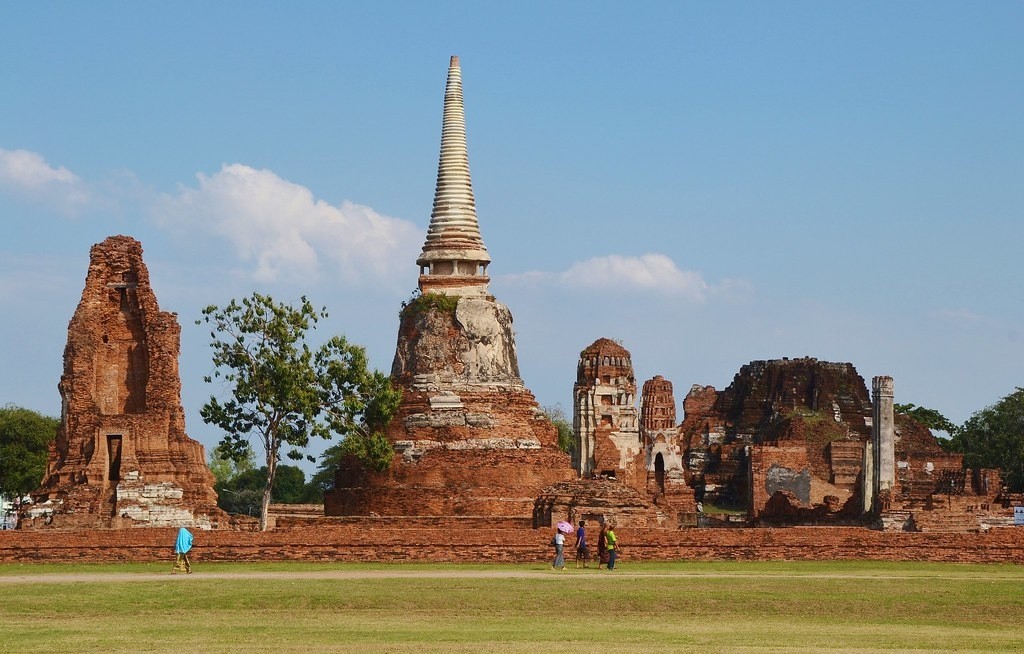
[550,537,556,546]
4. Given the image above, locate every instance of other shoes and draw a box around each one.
[562,567,567,571]
[551,566,556,571]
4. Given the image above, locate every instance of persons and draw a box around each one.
[550,528,567,570]
[575,521,589,568]
[171,528,194,575]
[606,525,618,570]
[598,523,610,569]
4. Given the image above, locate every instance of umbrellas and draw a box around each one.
[555,520,574,534]
